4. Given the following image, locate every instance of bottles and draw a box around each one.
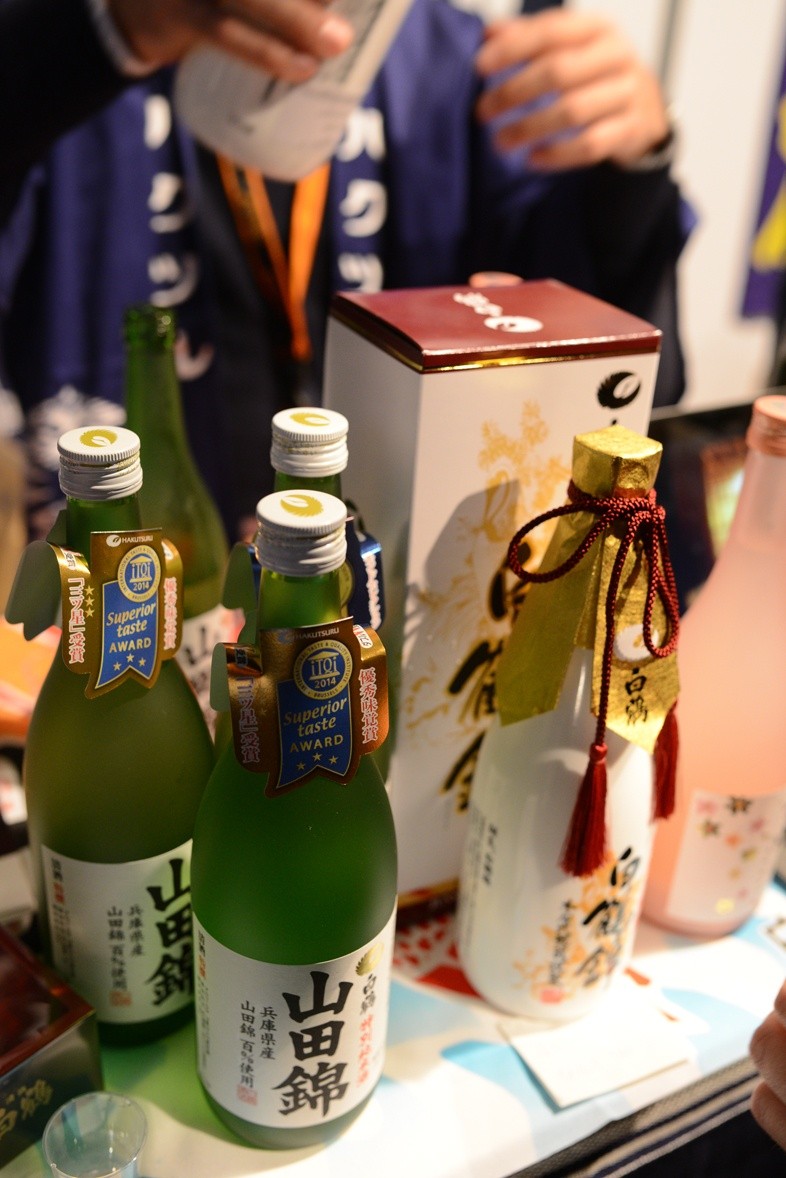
[213,408,369,764]
[22,426,214,1051]
[120,305,247,756]
[639,394,786,940]
[190,489,401,1150]
[450,428,676,1021]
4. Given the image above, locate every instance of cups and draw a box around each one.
[41,1090,148,1178]
[174,1,423,184]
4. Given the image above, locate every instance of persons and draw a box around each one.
[0,3,696,551]
